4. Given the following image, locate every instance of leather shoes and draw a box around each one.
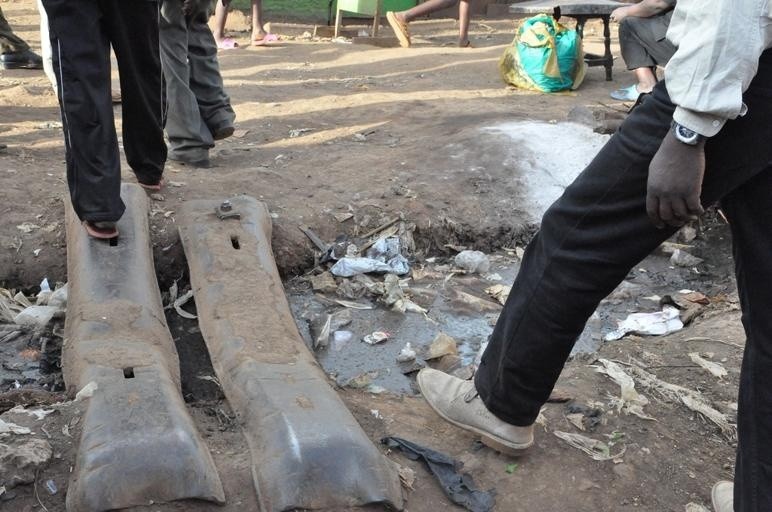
[711,479,736,512]
[416,366,536,459]
[1,50,43,68]
[212,120,236,142]
[167,147,210,168]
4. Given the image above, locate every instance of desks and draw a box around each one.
[509,0,634,81]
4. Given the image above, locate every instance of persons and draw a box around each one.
[0,5,44,68]
[386,0,476,48]
[211,0,284,49]
[41,0,169,241]
[157,0,237,170]
[416,0,770,512]
[610,0,679,102]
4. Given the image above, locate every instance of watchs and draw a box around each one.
[670,120,707,146]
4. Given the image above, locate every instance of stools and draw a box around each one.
[656,64,665,81]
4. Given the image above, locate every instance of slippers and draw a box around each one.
[82,217,122,241]
[218,38,241,49]
[387,10,412,48]
[610,85,649,101]
[251,34,289,49]
[138,179,167,192]
